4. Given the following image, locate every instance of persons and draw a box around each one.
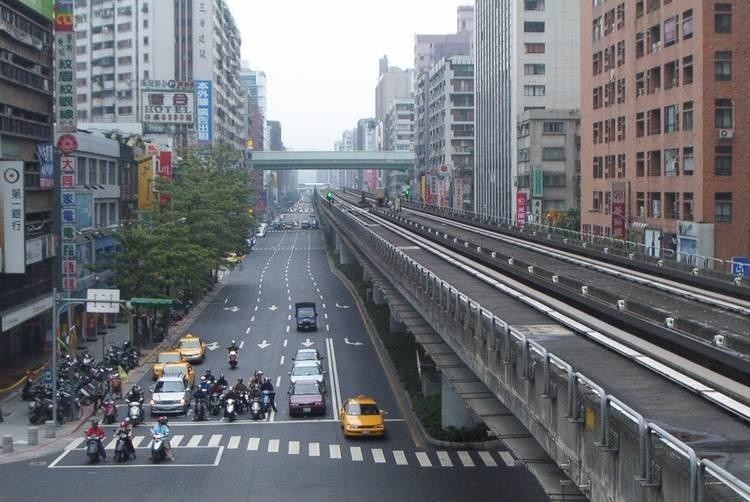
[188,366,277,421]
[82,353,176,463]
[228,340,239,353]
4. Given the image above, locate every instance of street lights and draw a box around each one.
[50,286,173,421]
[588,207,632,248]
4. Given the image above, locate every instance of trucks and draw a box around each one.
[295,302,318,332]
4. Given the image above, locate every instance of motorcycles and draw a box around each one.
[228,351,238,368]
[103,341,139,379]
[195,381,275,423]
[86,430,167,463]
[21,353,103,426]
[103,398,144,426]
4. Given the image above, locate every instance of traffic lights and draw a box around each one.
[405,191,409,195]
[327,192,333,200]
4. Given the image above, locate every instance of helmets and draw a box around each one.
[158,416,168,424]
[92,419,98,425]
[198,369,271,392]
[232,341,235,345]
[120,417,130,427]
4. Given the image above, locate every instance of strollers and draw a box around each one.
[110,378,122,398]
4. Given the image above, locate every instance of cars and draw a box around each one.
[245,222,266,247]
[340,395,385,440]
[221,252,238,263]
[287,206,315,213]
[150,334,206,416]
[275,220,319,230]
[287,348,326,418]
